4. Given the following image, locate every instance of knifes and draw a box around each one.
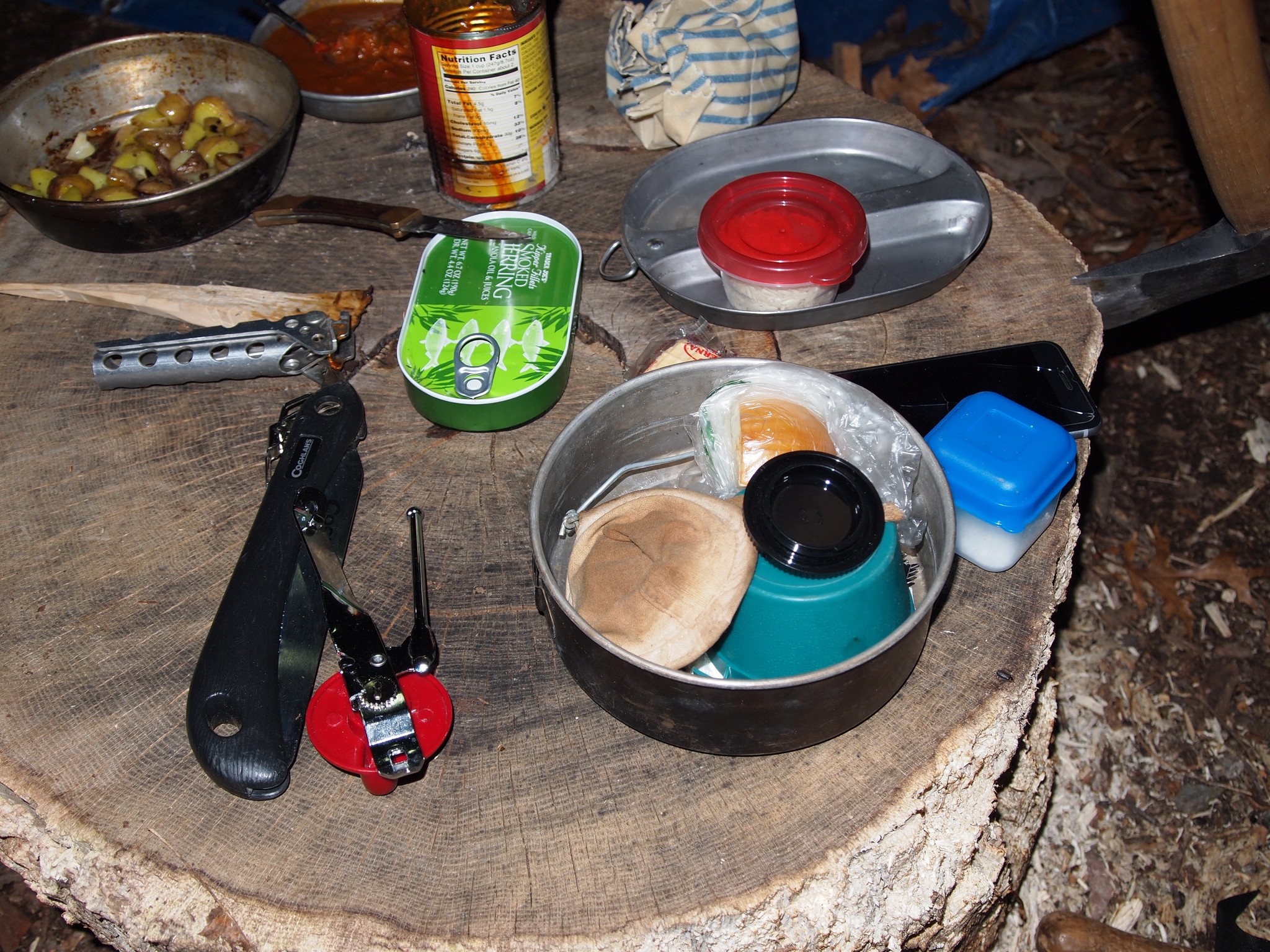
[252,193,532,240]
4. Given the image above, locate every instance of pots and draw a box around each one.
[0,32,302,255]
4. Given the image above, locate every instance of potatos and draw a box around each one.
[10,91,266,202]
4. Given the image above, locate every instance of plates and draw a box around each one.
[251,0,421,125]
[599,116,993,331]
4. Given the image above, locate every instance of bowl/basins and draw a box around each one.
[527,357,957,758]
[697,170,869,311]
[707,519,916,680]
